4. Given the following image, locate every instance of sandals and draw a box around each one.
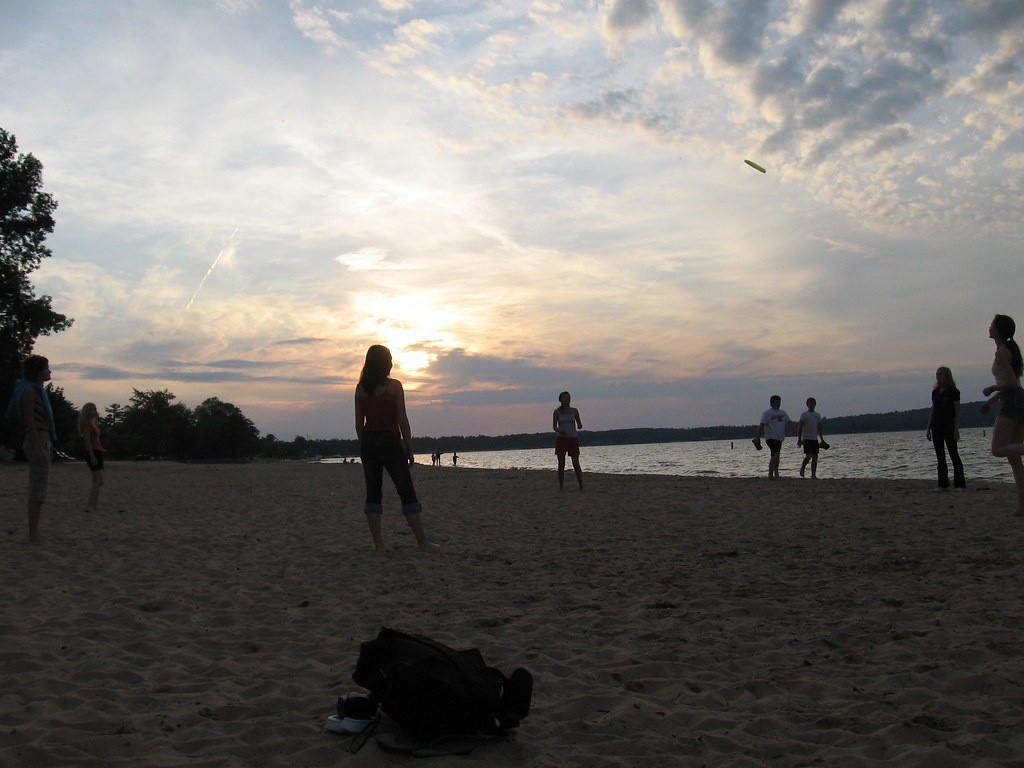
[325,692,377,734]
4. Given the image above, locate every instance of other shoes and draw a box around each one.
[954,487,967,492]
[932,487,947,493]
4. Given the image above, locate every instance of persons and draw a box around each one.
[432,448,443,467]
[20,355,51,544]
[756,395,797,480]
[354,345,441,556]
[553,391,584,492]
[79,403,107,513]
[926,367,967,492]
[979,314,1024,517]
[797,398,826,479]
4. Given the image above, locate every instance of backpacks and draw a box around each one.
[350,627,532,754]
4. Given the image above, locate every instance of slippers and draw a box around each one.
[375,727,511,757]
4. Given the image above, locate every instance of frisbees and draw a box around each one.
[744,160,766,174]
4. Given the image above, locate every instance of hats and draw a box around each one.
[559,392,570,401]
[23,355,48,375]
